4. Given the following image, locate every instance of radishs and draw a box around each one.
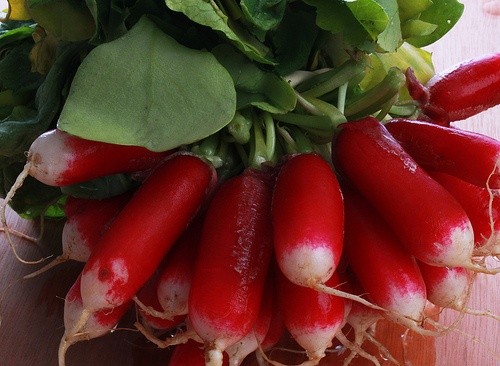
[1,1,500,366]
